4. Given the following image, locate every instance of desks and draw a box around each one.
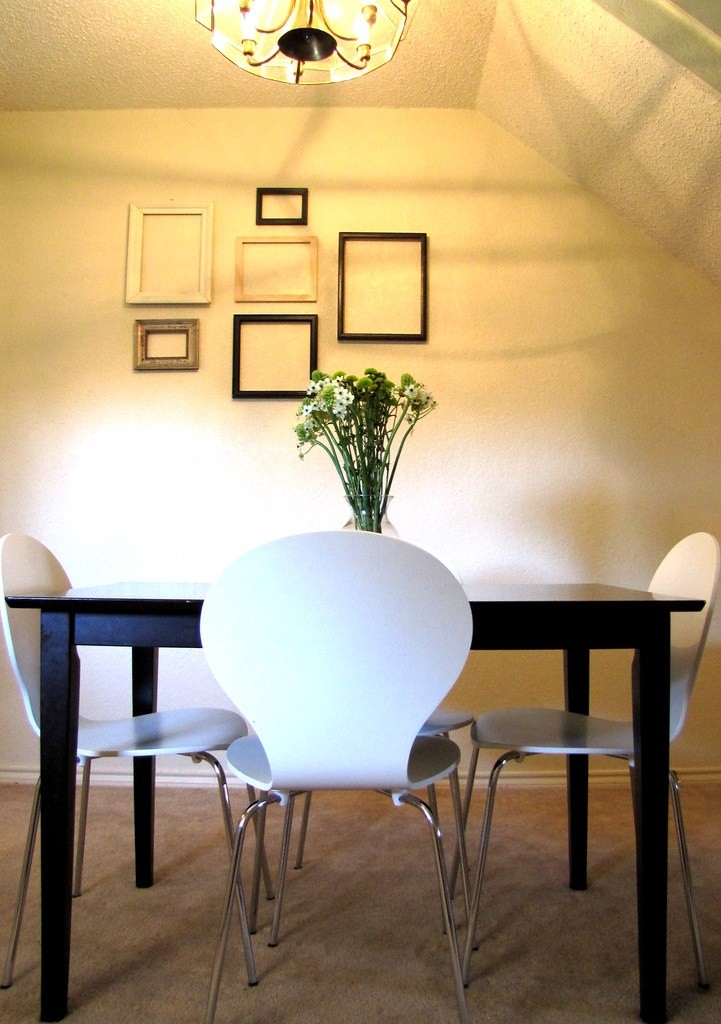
[4,582,706,1023]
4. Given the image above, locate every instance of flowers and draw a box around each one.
[294,367,438,532]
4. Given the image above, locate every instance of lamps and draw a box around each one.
[195,1,411,86]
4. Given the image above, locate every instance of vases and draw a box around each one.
[342,493,394,536]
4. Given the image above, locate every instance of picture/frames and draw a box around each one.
[134,318,200,370]
[236,235,320,303]
[125,200,213,305]
[255,187,308,227]
[233,313,318,399]
[337,232,426,342]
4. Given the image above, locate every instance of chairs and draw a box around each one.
[0,531,274,991]
[200,529,478,1021]
[446,528,720,991]
